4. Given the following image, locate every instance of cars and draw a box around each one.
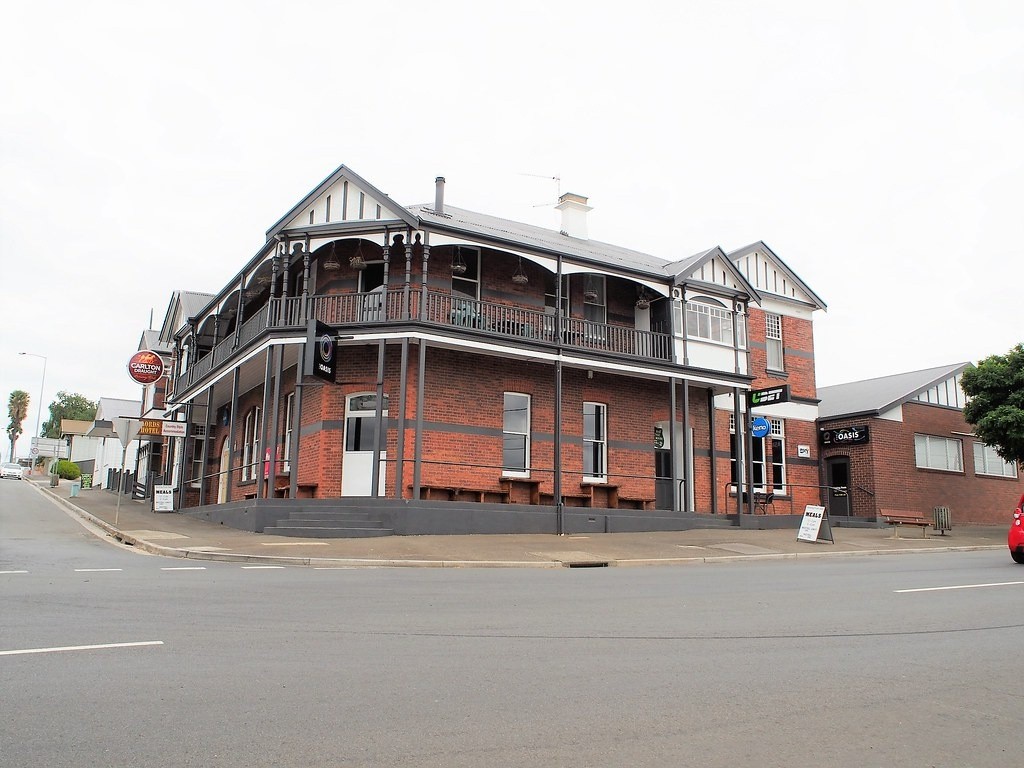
[1007,492,1024,564]
[0,461,28,480]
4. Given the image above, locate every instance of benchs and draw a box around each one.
[407,484,509,503]
[880,509,936,539]
[620,496,657,510]
[243,492,257,499]
[540,492,591,507]
[276,482,317,498]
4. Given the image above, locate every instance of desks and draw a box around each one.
[263,474,289,498]
[581,482,619,507]
[561,330,584,345]
[753,493,766,514]
[494,319,524,336]
[498,476,545,505]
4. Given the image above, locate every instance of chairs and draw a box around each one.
[742,492,748,514]
[755,493,775,514]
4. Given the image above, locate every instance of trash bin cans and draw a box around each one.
[50,474,60,486]
[933,506,952,530]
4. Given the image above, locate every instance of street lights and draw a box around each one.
[18,351,49,475]
